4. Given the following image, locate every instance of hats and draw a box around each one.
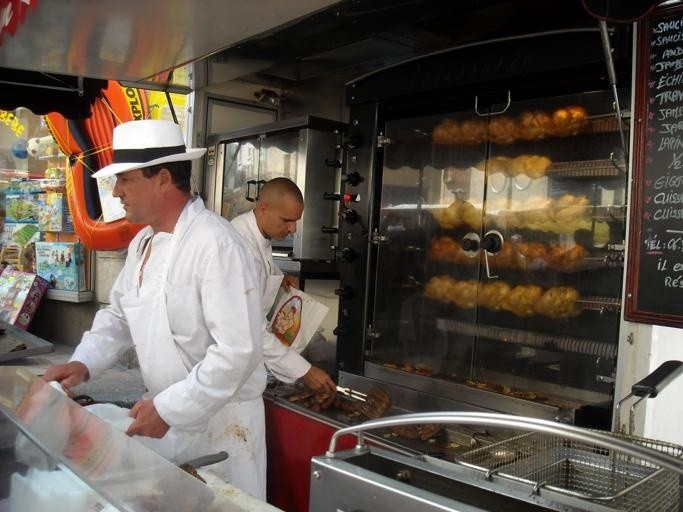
[92,119,209,179]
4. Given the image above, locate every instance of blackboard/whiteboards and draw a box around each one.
[624,2,683,331]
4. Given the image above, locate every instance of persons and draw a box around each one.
[226,177,338,400]
[36,117,268,504]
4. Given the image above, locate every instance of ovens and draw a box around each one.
[332,27,653,438]
[201,115,347,273]
